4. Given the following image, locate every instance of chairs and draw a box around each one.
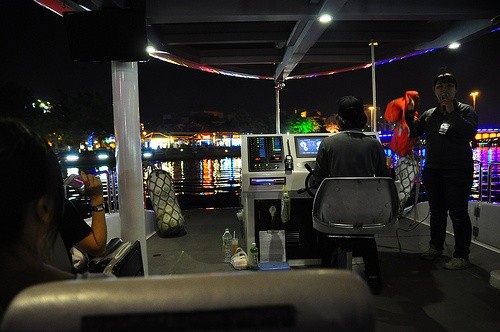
[312,178,401,239]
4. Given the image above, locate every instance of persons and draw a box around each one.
[314,96,388,294]
[49,171,107,272]
[405,72,478,270]
[0,120,75,322]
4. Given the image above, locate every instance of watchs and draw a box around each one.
[92,204,104,212]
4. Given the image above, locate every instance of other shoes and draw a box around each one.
[365,276,382,296]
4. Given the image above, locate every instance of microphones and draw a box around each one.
[441,93,449,114]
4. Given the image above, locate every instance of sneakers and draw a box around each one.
[443,256,470,270]
[421,248,442,260]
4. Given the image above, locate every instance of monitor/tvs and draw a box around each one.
[292,134,331,158]
[244,137,287,171]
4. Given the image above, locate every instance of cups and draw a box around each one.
[231,238,239,256]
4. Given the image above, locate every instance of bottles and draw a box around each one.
[249,243,258,271]
[224,239,233,263]
[222,228,232,256]
[281,193,290,222]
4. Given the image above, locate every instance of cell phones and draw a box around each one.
[62,174,85,189]
